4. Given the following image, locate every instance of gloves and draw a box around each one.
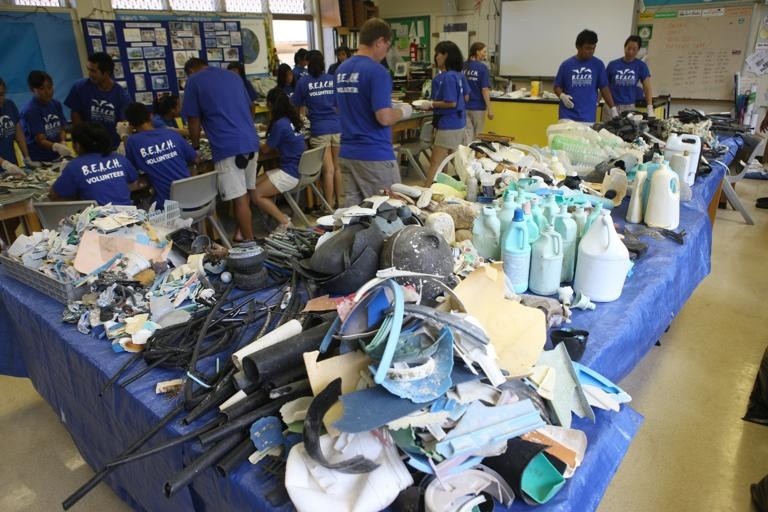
[647,104,655,118]
[2,160,26,176]
[560,93,574,108]
[52,143,71,156]
[397,101,412,119]
[419,101,434,110]
[610,106,618,118]
[116,121,130,138]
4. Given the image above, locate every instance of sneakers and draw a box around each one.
[311,210,329,216]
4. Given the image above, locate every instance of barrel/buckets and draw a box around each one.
[571,208,629,303]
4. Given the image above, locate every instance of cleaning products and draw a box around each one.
[473,186,603,291]
[626,132,701,230]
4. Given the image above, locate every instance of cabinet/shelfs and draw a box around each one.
[317,0,379,37]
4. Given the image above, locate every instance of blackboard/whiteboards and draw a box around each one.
[496,0,640,82]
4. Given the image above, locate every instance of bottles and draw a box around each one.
[531,82,540,99]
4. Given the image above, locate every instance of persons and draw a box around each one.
[151,94,202,137]
[554,29,619,124]
[0,52,131,178]
[47,120,139,206]
[227,46,353,233]
[755,109,768,209]
[333,18,415,211]
[414,39,472,188]
[462,42,493,139]
[596,33,655,124]
[124,102,203,212]
[182,58,261,245]
[428,52,457,177]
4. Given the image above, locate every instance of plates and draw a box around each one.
[411,100,429,108]
